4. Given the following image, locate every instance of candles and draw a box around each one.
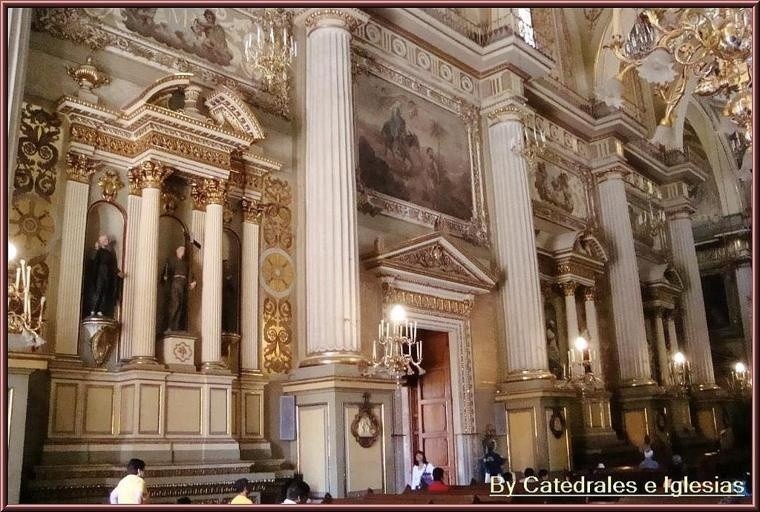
[726,369,752,384]
[567,348,592,364]
[7,260,47,321]
[372,319,423,361]
[668,361,691,372]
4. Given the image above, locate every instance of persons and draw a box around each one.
[410,449,437,491]
[425,467,449,493]
[85,234,128,320]
[502,471,512,480]
[421,147,442,211]
[109,458,147,504]
[547,319,560,370]
[226,477,254,504]
[591,456,609,474]
[638,446,658,470]
[638,435,651,462]
[536,468,549,482]
[380,106,407,161]
[667,453,689,480]
[518,467,537,483]
[279,480,310,505]
[190,10,234,66]
[157,245,197,336]
[482,438,506,477]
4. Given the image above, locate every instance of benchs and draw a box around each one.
[318,479,751,504]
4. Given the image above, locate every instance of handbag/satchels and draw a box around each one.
[419,473,432,489]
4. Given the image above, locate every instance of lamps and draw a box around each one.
[601,8,752,145]
